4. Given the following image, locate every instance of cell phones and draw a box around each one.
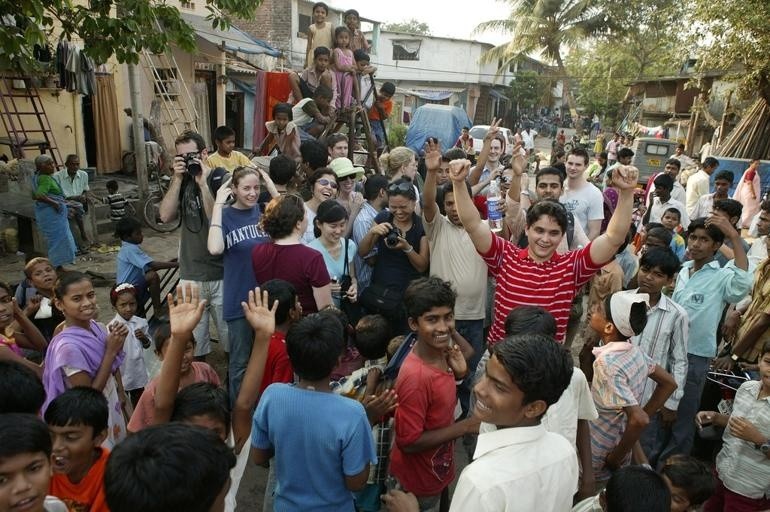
[224,194,235,204]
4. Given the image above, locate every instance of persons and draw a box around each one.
[289,46,337,113]
[369,82,396,149]
[343,9,372,53]
[252,102,303,162]
[0,108,770,510]
[329,27,359,109]
[354,48,377,101]
[303,2,334,69]
[292,86,334,143]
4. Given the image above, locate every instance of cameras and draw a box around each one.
[385,227,403,247]
[176,152,201,176]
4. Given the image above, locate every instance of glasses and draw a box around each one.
[500,176,513,183]
[530,202,565,213]
[316,179,339,188]
[330,132,346,139]
[387,182,413,193]
[338,173,356,181]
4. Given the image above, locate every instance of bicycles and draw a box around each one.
[143,160,182,234]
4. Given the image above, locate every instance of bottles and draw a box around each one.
[486,180,504,233]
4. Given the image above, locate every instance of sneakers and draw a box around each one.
[79,246,89,253]
[74,246,81,257]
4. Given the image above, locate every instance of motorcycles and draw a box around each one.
[563,136,597,154]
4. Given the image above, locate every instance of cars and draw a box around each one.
[469,125,516,169]
[628,137,678,191]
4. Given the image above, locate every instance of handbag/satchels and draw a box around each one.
[341,236,362,316]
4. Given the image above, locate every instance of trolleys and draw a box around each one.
[122,108,158,178]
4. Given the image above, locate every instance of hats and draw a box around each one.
[326,156,366,183]
